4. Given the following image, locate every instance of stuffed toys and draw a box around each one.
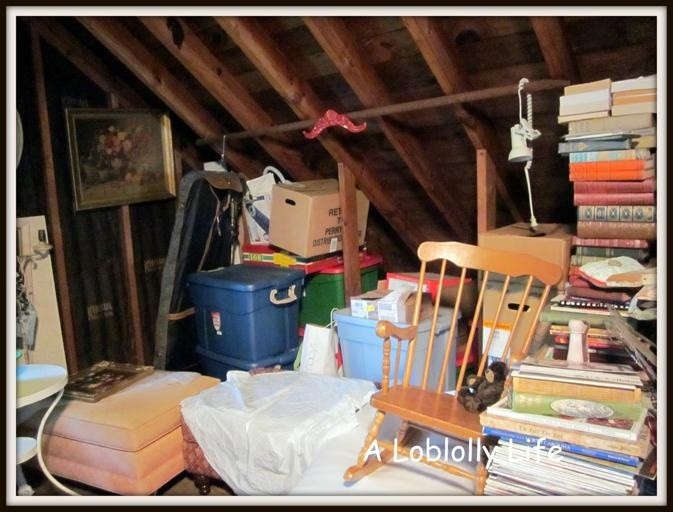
[456,362,507,415]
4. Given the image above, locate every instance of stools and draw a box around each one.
[179,367,377,496]
[20,366,222,496]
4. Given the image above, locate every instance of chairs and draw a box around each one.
[343,241,563,496]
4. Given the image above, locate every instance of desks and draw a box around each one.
[15,363,84,496]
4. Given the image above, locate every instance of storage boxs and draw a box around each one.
[477,222,578,376]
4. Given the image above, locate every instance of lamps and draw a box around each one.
[507,77,542,228]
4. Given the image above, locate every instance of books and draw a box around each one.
[476,74,657,496]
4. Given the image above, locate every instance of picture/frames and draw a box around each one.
[54,360,155,403]
[63,104,178,211]
